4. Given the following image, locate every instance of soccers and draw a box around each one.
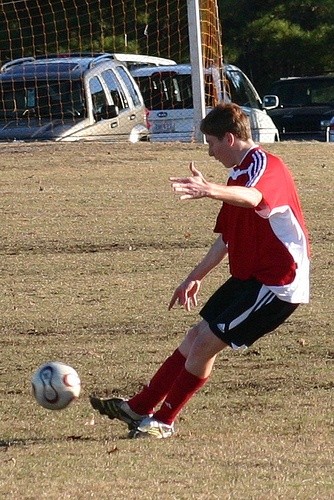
[31,361,81,410]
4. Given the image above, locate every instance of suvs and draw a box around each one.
[0,54,151,143]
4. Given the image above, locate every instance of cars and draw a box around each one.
[264,73,334,143]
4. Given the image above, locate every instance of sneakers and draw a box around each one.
[133,418,176,440]
[89,397,147,432]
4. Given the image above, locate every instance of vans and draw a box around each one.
[132,65,280,143]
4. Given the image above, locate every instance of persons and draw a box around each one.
[89,104,310,440]
